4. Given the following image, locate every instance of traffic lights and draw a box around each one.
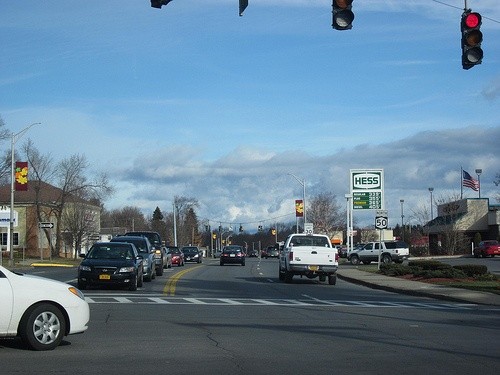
[332,0,355,31]
[459,11,485,70]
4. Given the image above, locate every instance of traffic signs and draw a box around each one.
[37,221,54,229]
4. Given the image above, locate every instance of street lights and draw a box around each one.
[475,169,483,198]
[10,121,41,268]
[287,171,307,232]
[428,186,435,221]
[400,199,405,227]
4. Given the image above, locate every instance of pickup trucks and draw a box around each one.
[345,241,410,264]
[279,233,339,285]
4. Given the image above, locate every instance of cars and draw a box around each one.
[114,240,157,282]
[182,246,202,263]
[0,263,90,349]
[220,244,246,267]
[473,240,500,258]
[332,243,351,258]
[249,249,268,257]
[163,247,173,268]
[78,242,143,290]
[125,231,164,276]
[265,245,281,257]
[166,246,184,266]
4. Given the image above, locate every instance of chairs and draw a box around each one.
[92,249,106,259]
